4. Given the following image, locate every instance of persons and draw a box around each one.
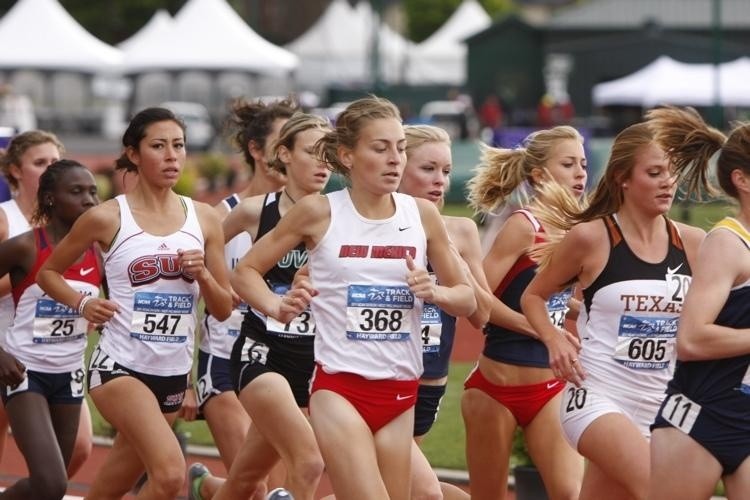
[0,91,750,500]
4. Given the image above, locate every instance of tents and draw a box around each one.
[0,0,750,108]
